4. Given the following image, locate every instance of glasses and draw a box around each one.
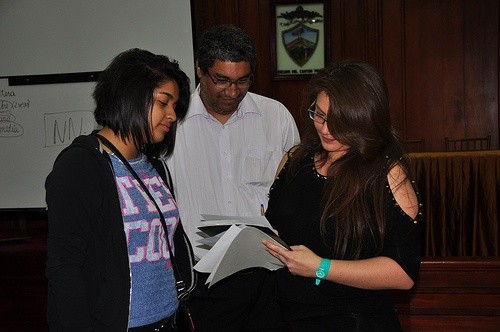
[308,100,327,124]
[207,71,251,89]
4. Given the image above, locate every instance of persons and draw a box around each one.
[261,60,424,332]
[142,24,301,332]
[45,48,198,332]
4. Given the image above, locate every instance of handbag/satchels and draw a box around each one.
[173,220,198,299]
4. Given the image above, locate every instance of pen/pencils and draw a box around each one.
[259,202,266,219]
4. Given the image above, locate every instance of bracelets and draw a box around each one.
[315,258,331,285]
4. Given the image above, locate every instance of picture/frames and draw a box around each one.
[271,0,329,81]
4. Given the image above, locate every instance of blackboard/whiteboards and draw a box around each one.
[0,1,199,207]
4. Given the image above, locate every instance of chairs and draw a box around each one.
[431,135,492,257]
[396,139,424,194]
